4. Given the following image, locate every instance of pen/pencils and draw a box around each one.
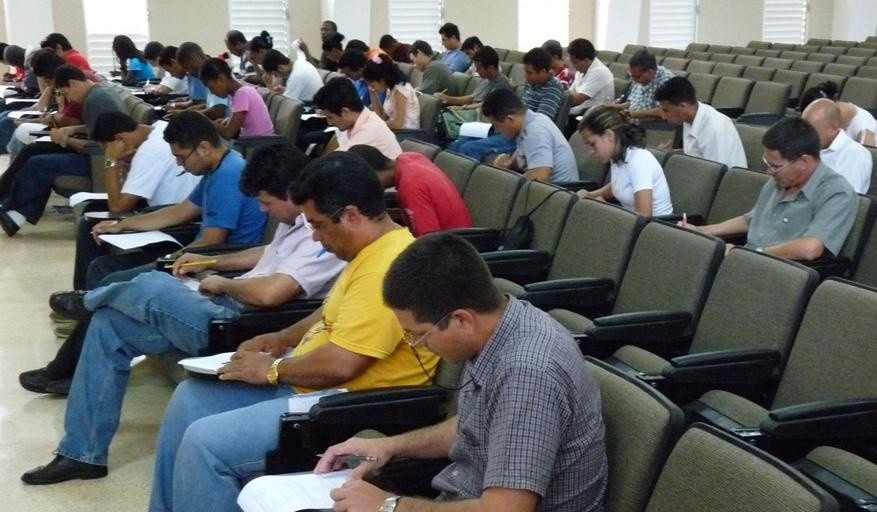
[89,216,125,234]
[436,88,449,96]
[307,451,380,463]
[221,360,234,365]
[50,116,58,131]
[682,213,688,227]
[161,261,216,269]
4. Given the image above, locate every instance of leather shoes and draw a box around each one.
[21,454,107,484]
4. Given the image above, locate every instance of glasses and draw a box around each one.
[762,151,800,174]
[402,310,455,348]
[177,148,195,166]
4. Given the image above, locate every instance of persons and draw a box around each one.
[597,48,678,128]
[796,81,876,148]
[655,75,747,169]
[571,101,674,223]
[1,19,615,507]
[799,97,873,197]
[675,117,859,263]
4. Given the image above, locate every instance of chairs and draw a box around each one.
[794,442,875,510]
[545,218,726,358]
[580,357,685,509]
[685,274,875,459]
[644,422,837,510]
[72,25,876,290]
[583,245,820,417]
[148,291,529,511]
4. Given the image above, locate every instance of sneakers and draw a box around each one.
[50,292,94,320]
[19,363,70,394]
[0,206,20,235]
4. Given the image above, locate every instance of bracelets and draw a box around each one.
[755,246,764,254]
[618,109,631,121]
